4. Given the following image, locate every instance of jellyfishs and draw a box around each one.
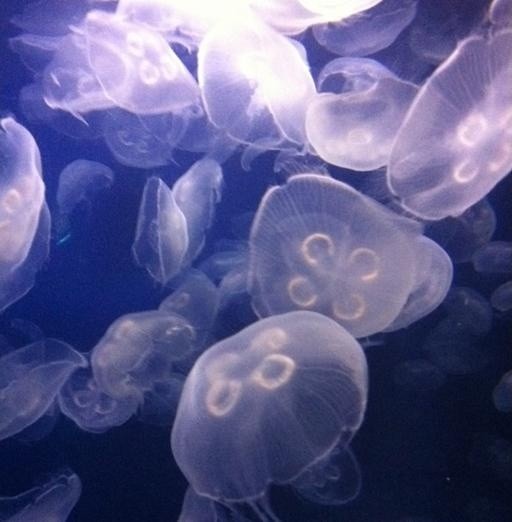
[1,1,512,522]
